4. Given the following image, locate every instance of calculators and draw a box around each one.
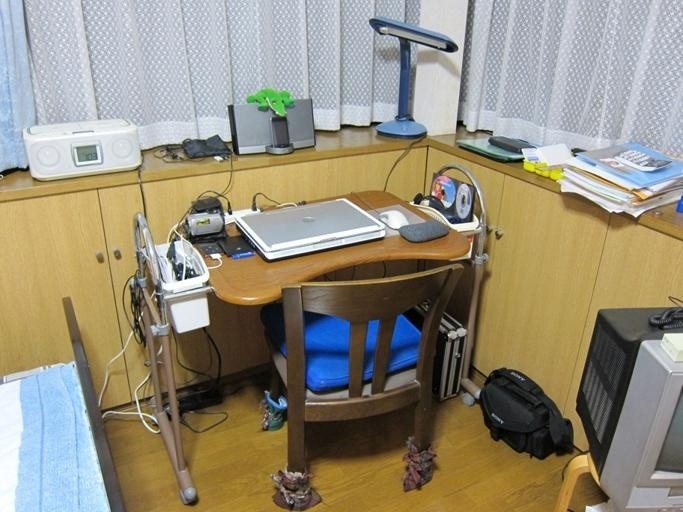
[613,149,672,172]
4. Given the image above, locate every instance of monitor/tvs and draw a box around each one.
[575,307,683,512]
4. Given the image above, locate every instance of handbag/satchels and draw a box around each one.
[480,368,573,461]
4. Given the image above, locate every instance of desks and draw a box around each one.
[132,163,488,506]
[553,452,600,512]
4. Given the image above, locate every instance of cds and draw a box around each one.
[430,175,456,210]
[456,184,472,219]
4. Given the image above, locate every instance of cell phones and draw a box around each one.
[198,244,225,258]
[270,116,289,148]
[218,236,254,258]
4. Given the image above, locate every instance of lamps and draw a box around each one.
[369,17,458,138]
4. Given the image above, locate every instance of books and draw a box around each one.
[559,143,683,218]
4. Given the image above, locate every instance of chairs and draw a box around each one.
[258,262,464,511]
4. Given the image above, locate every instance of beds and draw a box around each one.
[0,297,124,512]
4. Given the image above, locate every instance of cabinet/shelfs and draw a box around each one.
[425,147,610,419]
[0,148,427,412]
[564,213,682,452]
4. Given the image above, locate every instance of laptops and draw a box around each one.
[234,198,387,263]
